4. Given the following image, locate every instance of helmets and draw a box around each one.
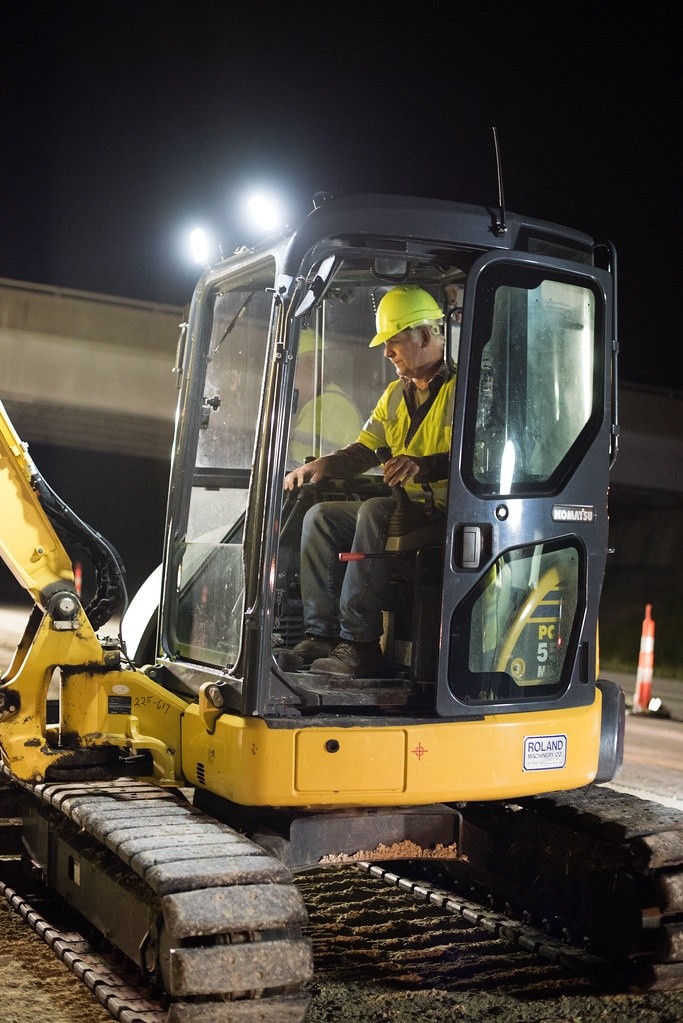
[369,285,446,348]
[297,327,330,354]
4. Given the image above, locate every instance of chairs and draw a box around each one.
[380,368,516,680]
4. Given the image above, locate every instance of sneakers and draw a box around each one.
[310,638,383,679]
[273,634,341,673]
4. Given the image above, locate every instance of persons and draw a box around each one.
[283,284,457,677]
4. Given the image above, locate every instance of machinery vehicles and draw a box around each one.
[1,188,682,1022]
